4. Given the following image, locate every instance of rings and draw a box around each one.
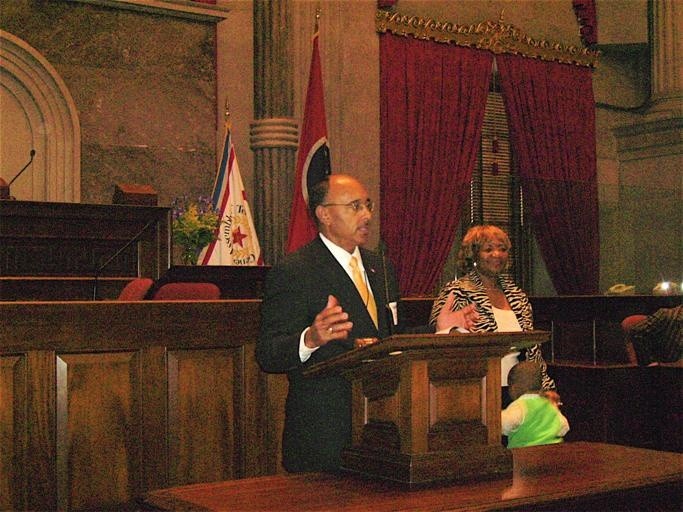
[327,326,333,333]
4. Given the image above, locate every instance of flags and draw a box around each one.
[287,2,331,253]
[197,95,264,267]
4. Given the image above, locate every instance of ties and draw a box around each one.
[349,255,379,328]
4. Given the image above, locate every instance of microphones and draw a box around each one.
[378,238,393,336]
[93,215,158,301]
[8,150,35,195]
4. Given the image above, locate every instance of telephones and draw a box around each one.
[605,283,635,295]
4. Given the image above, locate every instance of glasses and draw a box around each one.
[322,200,376,212]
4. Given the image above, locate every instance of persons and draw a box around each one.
[624,304,682,366]
[502,361,570,448]
[256,174,478,472]
[429,226,561,448]
